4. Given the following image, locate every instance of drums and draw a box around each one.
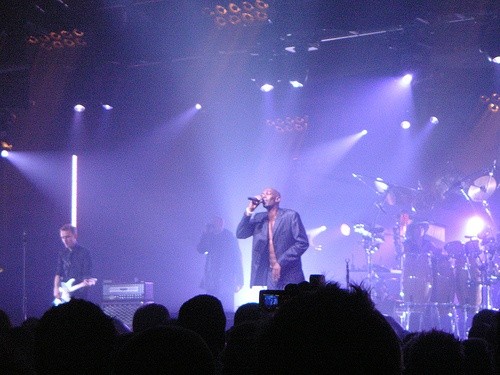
[404,239,482,307]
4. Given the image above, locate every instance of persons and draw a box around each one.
[195,216,244,315]
[235,188,309,289]
[53,224,91,302]
[403,215,454,335]
[0,279,500,375]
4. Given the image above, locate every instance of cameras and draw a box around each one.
[259,289,286,308]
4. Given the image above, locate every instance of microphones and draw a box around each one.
[248,197,264,202]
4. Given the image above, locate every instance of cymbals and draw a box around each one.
[468,175,497,203]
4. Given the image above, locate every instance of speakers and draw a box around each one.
[101,281,153,333]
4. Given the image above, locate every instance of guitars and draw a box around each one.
[52,276,98,306]
